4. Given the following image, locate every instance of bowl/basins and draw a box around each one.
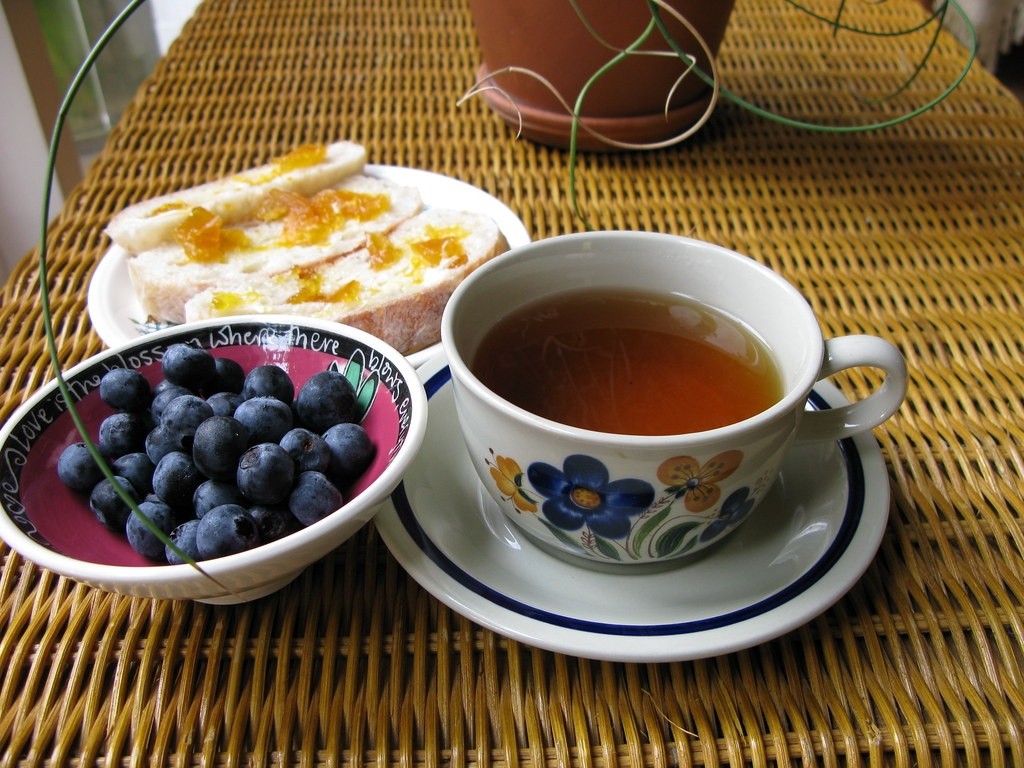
[1,313,429,606]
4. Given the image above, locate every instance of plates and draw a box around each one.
[371,348,891,666]
[86,163,532,370]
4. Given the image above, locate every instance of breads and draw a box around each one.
[106,141,508,359]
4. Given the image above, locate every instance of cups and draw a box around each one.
[439,229,910,565]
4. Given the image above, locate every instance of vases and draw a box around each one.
[465,1,738,117]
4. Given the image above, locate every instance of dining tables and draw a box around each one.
[0,0,1021,767]
